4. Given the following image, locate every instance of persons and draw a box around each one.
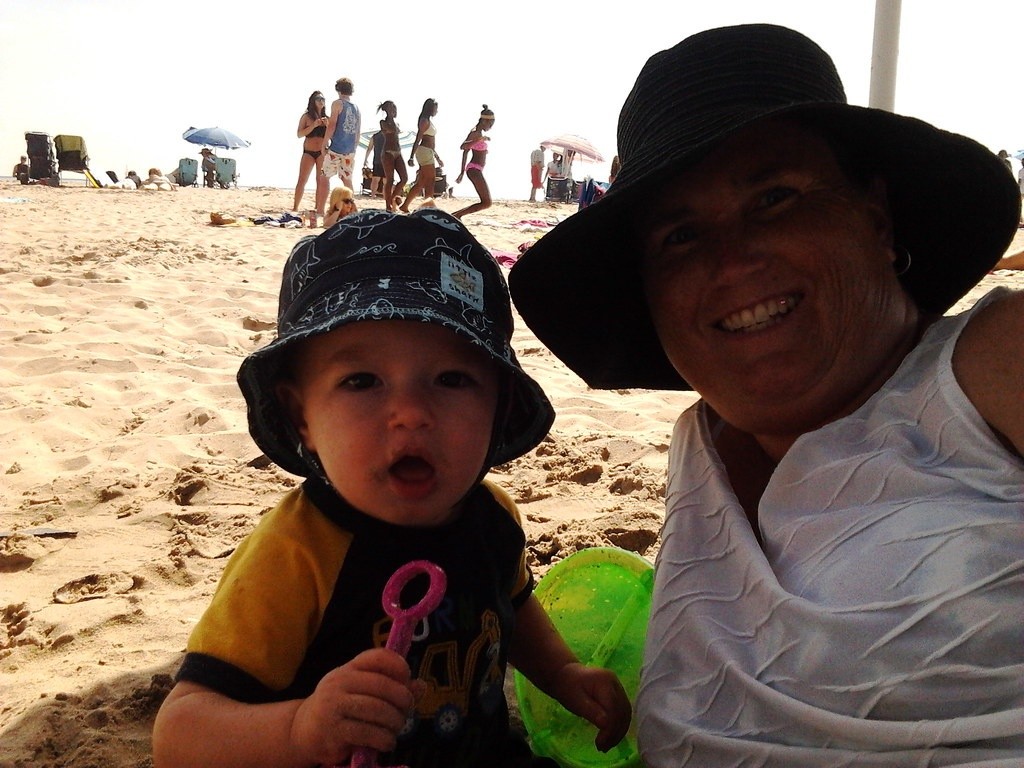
[141,168,172,191]
[507,24,1024,768]
[13,156,28,180]
[317,77,361,214]
[292,90,329,212]
[109,168,141,190]
[198,148,230,188]
[377,100,407,211]
[451,104,495,218]
[323,186,358,227]
[152,206,633,768]
[997,150,1024,224]
[364,129,385,199]
[529,146,559,202]
[399,98,444,212]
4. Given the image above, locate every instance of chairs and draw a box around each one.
[23,130,91,188]
[547,176,570,204]
[174,158,200,189]
[204,158,238,188]
[361,169,386,197]
[569,180,586,204]
[423,168,449,198]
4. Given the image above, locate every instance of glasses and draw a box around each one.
[343,198,353,204]
[315,97,325,101]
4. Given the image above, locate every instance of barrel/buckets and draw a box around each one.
[509,544,664,768]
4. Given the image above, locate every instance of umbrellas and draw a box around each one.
[358,124,416,149]
[540,133,608,179]
[183,127,251,149]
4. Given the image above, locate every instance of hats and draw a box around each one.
[199,148,212,154]
[237,211,554,479]
[508,24,1021,391]
